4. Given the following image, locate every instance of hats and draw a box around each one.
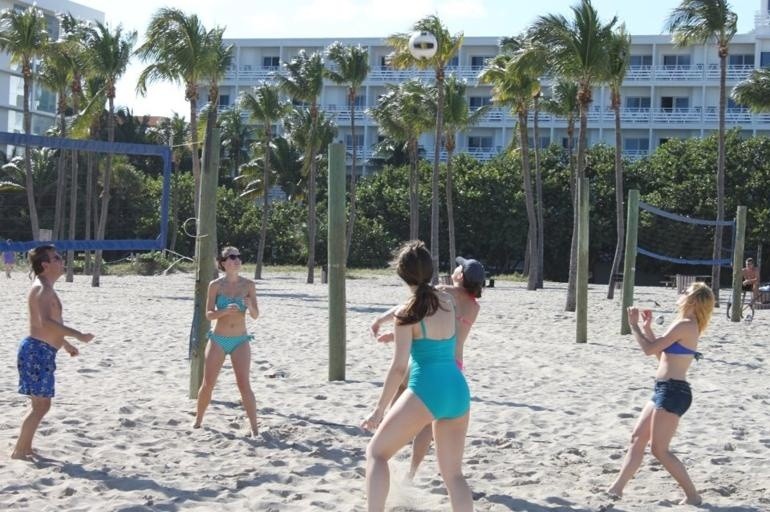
[456,256,484,284]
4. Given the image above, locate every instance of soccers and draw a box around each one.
[408,31,438,60]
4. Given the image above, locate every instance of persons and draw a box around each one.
[372,256,485,487]
[4,239,14,278]
[191,247,258,436]
[605,281,715,505]
[741,256,759,303]
[360,240,473,512]
[12,245,94,461]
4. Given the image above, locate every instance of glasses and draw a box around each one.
[224,255,243,260]
